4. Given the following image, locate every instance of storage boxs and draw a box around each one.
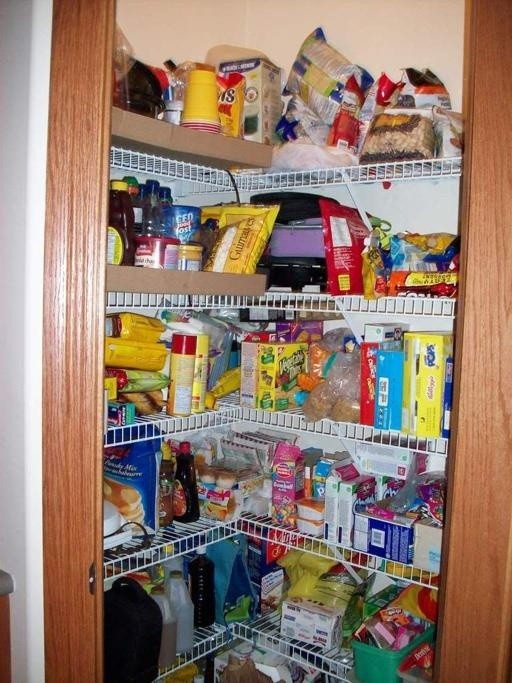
[111,106,274,169]
[214,626,290,682]
[350,602,434,683]
[106,263,268,296]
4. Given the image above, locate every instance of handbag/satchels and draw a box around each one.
[104,576,162,683]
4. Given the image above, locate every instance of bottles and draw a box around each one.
[156,442,200,526]
[150,543,216,666]
[106,176,175,268]
[167,331,209,417]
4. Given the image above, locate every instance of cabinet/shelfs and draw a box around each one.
[107,147,462,682]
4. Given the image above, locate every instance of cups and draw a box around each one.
[179,69,220,135]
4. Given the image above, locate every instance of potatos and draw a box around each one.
[302,383,361,424]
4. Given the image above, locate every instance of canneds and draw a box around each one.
[134,205,203,272]
